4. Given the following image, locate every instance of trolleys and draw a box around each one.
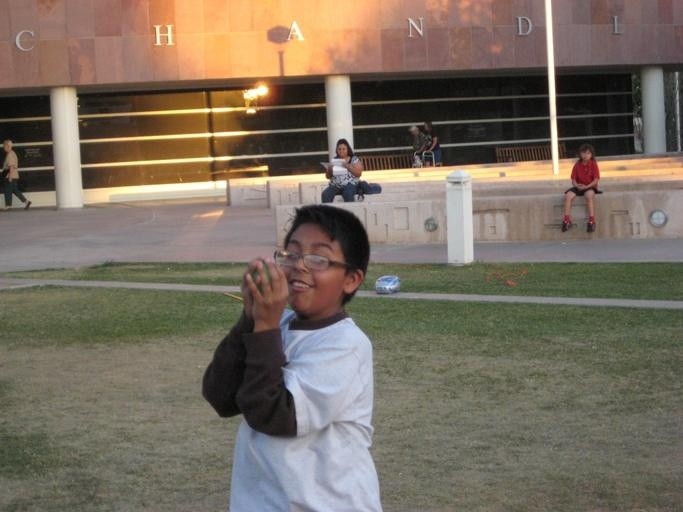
[412,150,435,168]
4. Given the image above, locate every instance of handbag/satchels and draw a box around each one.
[358,181,371,201]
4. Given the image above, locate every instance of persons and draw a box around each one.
[408,126,431,166]
[415,119,442,162]
[200,202,383,512]
[320,138,363,202]
[561,144,603,233]
[0,139,31,210]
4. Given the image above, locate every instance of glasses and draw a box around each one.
[274,249,352,271]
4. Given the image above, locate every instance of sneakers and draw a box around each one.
[562,221,571,232]
[587,222,594,232]
